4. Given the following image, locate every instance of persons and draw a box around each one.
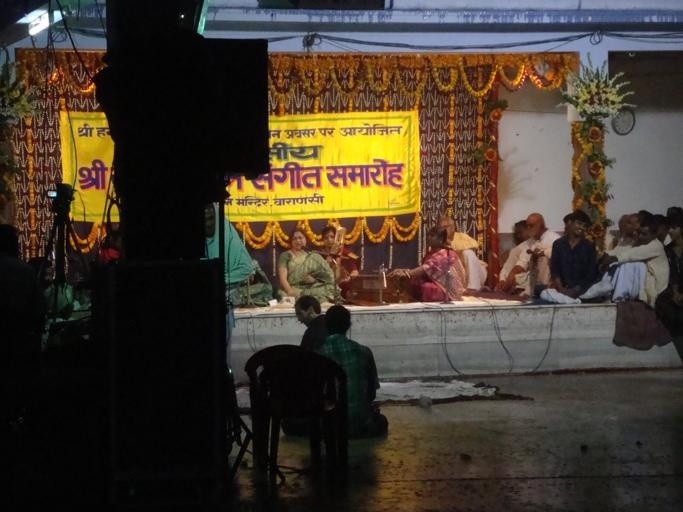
[313,304,388,440]
[0,194,46,510]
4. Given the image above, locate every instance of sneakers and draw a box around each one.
[367,405,390,428]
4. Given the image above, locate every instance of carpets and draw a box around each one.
[233,376,535,417]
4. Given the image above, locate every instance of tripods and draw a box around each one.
[32,211,91,355]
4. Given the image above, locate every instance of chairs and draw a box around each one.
[242,345,349,484]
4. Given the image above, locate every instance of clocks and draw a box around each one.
[610,108,636,135]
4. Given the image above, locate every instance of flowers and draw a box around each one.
[555,52,639,116]
[569,118,616,259]
[469,100,509,165]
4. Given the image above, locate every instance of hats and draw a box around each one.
[570,208,592,223]
